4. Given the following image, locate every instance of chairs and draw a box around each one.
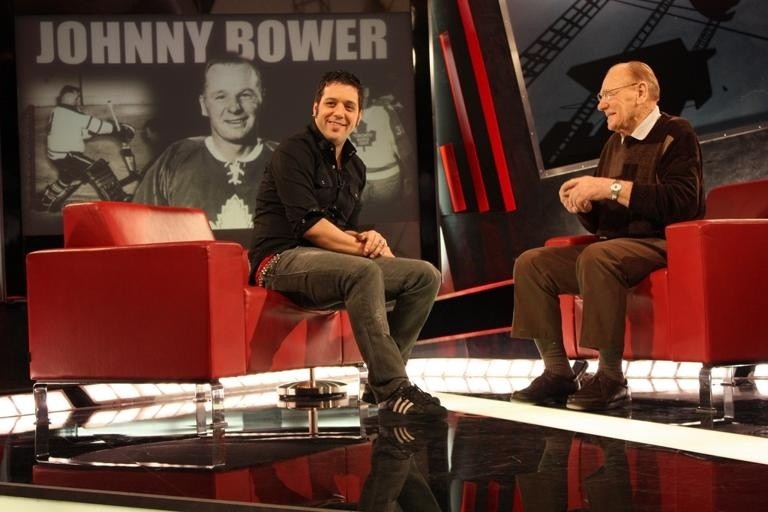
[33,395,377,507]
[568,412,768,512]
[25,200,363,423]
[545,179,768,413]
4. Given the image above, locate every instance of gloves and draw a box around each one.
[107,119,136,144]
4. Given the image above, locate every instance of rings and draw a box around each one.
[378,239,385,246]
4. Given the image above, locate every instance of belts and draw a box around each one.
[254,254,275,287]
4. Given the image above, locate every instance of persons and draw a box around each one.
[127,48,280,232]
[345,76,415,259]
[32,84,137,213]
[507,57,707,412]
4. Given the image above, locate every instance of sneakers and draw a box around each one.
[362,383,440,406]
[377,384,448,421]
[378,419,448,458]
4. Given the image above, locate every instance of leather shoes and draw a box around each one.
[566,369,632,411]
[509,368,579,404]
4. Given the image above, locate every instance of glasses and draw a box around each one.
[594,82,635,101]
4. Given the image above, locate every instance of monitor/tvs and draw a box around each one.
[0,1,443,304]
[499,0,768,182]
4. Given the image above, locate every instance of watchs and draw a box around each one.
[246,65,450,426]
[609,178,621,203]
